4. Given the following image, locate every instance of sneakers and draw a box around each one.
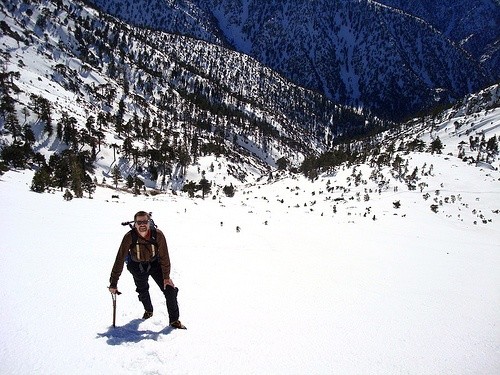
[169,320,187,329]
[143,311,153,319]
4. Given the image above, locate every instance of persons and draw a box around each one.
[109,211,187,330]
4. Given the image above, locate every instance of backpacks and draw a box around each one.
[121,211,158,248]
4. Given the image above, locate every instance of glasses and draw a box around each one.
[135,221,148,224]
